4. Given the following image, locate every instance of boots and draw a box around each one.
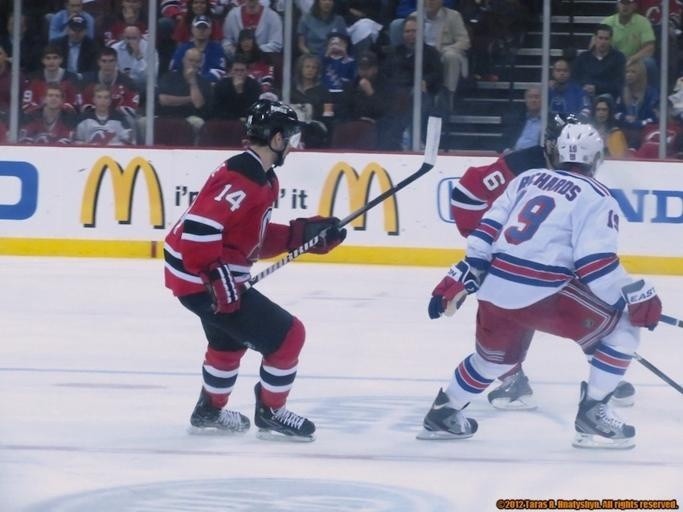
[487,364,534,402]
[253,383,316,437]
[607,376,639,397]
[575,380,638,440]
[189,390,251,433]
[425,388,481,436]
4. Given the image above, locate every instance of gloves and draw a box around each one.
[618,276,663,332]
[202,263,242,315]
[286,215,348,255]
[427,259,482,323]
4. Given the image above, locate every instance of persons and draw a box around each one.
[416,122,661,450]
[161,101,345,443]
[1,0,682,163]
[450,112,639,411]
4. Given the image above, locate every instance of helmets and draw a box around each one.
[545,113,582,150]
[556,124,606,166]
[241,97,304,144]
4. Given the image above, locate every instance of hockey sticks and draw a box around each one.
[212,116,441,311]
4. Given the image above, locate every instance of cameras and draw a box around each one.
[332,48,341,54]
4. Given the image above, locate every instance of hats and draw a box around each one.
[68,15,88,29]
[159,1,180,15]
[327,27,350,42]
[192,15,213,29]
